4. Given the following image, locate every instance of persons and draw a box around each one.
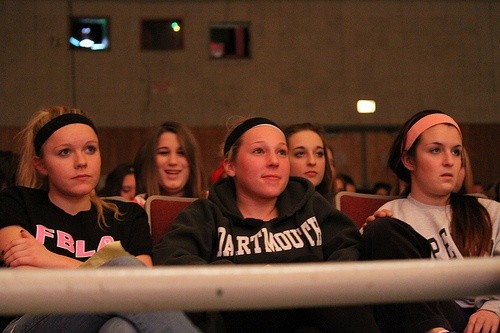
[0,120,500,208]
[0,104,202,333]
[360,109,500,333]
[152,116,376,333]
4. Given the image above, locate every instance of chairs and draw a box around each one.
[146,196,199,242]
[333,191,407,232]
